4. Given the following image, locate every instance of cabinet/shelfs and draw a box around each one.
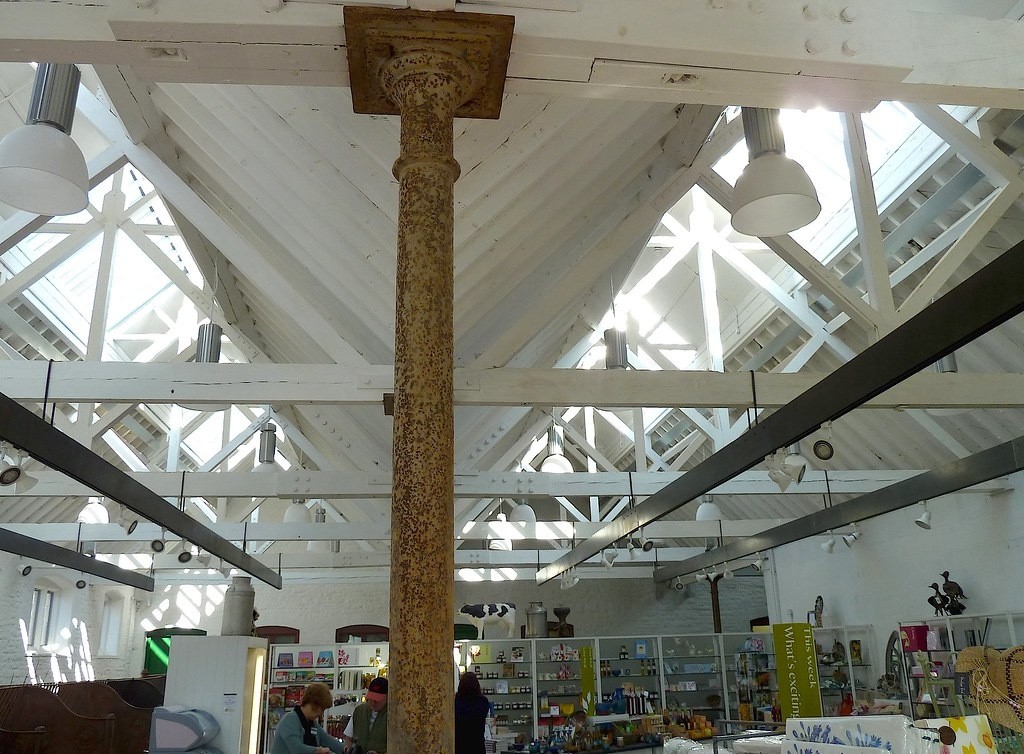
[258,622,877,754]
[897,610,1023,722]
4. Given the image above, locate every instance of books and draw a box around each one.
[341,670,357,690]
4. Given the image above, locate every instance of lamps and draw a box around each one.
[251,403,330,554]
[14,555,31,576]
[763,448,807,494]
[674,550,764,592]
[695,442,723,522]
[116,503,233,579]
[0,59,89,216]
[915,498,931,530]
[819,520,863,554]
[0,441,39,494]
[75,571,87,589]
[539,408,573,475]
[730,105,821,239]
[489,460,536,551]
[559,526,654,590]
[589,271,642,412]
[173,253,232,410]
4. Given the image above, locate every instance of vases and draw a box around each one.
[612,687,627,715]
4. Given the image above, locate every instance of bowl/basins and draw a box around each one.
[621,669,630,676]
[611,669,620,676]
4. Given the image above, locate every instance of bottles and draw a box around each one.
[374,648,382,667]
[678,707,697,731]
[601,645,656,677]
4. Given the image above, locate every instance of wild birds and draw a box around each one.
[928,570,967,616]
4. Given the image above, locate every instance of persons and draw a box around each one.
[455,672,490,754]
[270,682,348,754]
[343,677,387,754]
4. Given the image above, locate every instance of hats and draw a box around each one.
[366,677,388,702]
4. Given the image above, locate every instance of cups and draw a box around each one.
[617,737,625,747]
[369,657,375,666]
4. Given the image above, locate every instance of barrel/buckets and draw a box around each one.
[524,601,548,638]
[221,577,255,636]
[899,625,928,652]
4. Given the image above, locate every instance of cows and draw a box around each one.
[457,602,517,639]
